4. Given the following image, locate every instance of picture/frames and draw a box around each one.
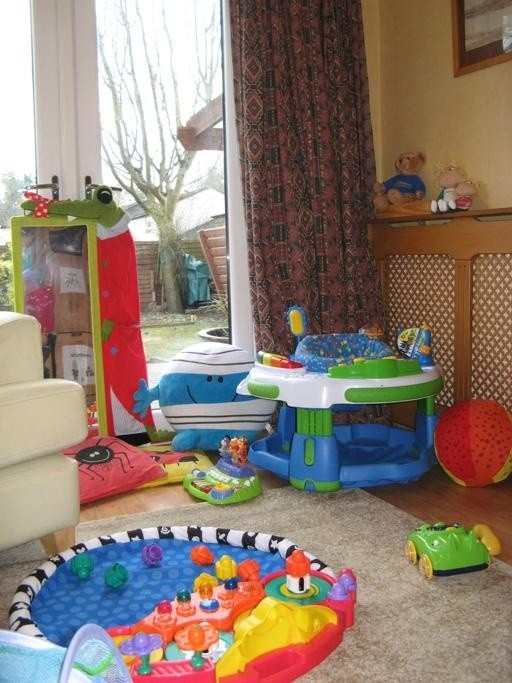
[451,0,512,78]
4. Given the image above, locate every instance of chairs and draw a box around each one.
[196,227,228,313]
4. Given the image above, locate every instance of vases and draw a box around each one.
[198,327,230,343]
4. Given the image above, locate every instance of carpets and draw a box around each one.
[0,487,512,683]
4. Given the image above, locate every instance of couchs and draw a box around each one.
[0,310,88,556]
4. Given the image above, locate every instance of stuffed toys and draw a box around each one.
[430,159,465,213]
[373,151,426,213]
[449,178,481,212]
[20,185,176,445]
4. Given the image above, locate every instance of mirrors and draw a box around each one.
[11,216,108,442]
